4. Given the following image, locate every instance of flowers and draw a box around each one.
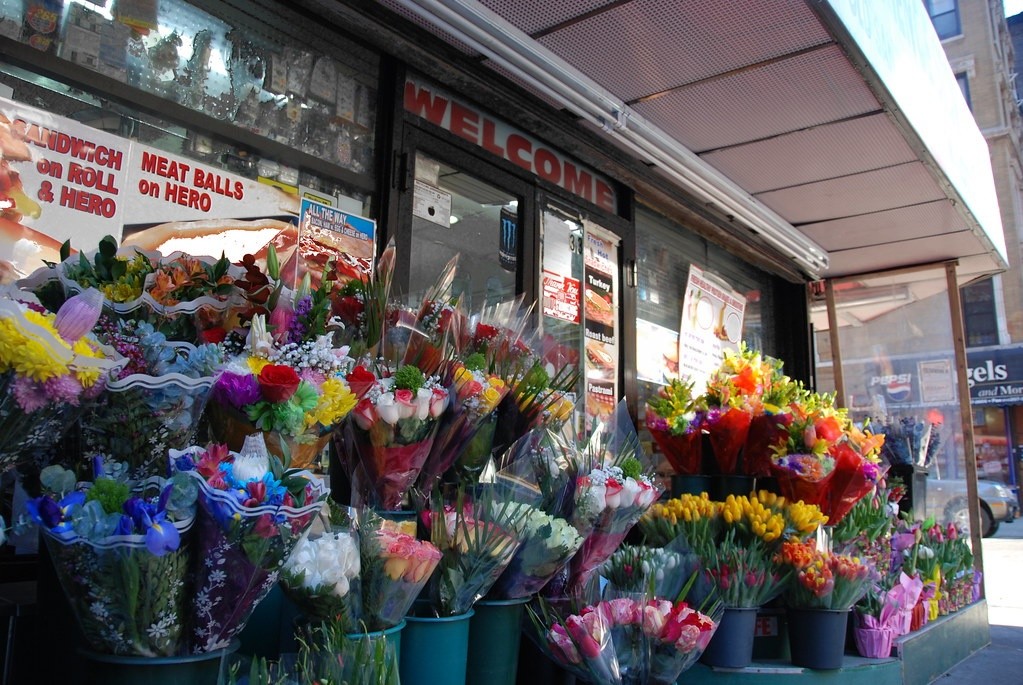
[0,235,975,685]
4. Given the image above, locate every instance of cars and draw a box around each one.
[915,479,1021,539]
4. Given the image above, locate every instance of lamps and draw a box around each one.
[393,0,832,279]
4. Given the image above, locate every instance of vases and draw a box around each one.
[897,611,911,635]
[927,598,940,621]
[715,473,754,503]
[85,638,241,685]
[400,599,475,685]
[516,593,580,685]
[701,605,761,668]
[920,600,931,624]
[939,590,950,616]
[753,475,782,499]
[668,474,713,499]
[787,605,849,672]
[947,586,960,612]
[887,613,900,638]
[466,595,534,685]
[956,587,965,607]
[910,603,924,631]
[331,617,405,685]
[964,584,973,604]
[856,627,893,659]
